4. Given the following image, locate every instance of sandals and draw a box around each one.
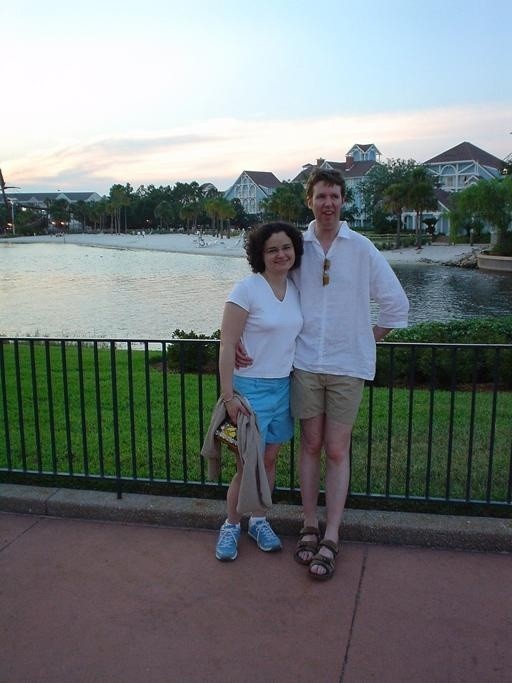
[308,538,340,581]
[294,525,322,566]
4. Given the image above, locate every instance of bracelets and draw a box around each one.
[222,392,233,404]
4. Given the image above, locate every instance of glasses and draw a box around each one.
[322,257,331,288]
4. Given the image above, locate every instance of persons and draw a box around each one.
[216,219,304,561]
[235,168,410,580]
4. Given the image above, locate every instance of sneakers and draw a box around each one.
[215,518,241,561]
[247,515,282,553]
